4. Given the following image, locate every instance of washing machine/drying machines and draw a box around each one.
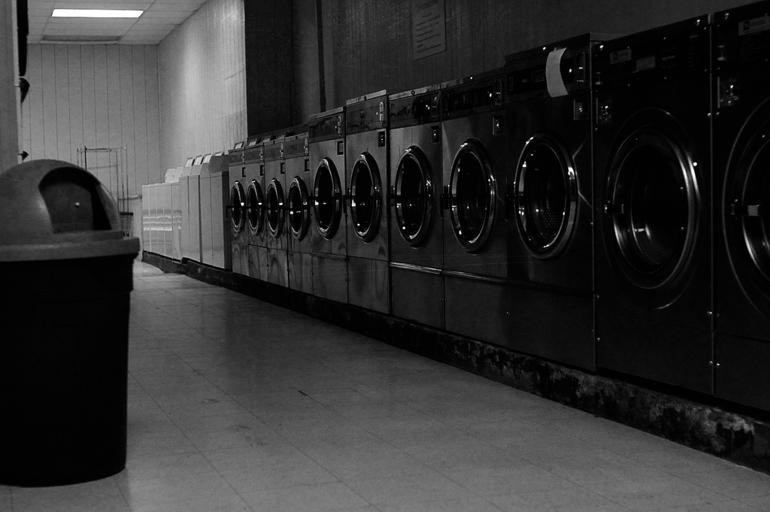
[140,0,770,417]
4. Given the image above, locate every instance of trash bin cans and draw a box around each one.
[0,159,140,488]
